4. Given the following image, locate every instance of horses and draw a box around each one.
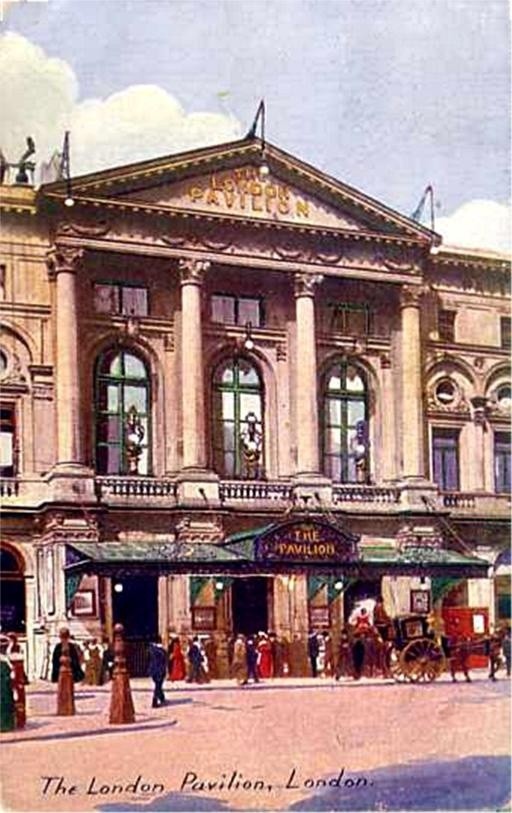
[449,618,508,683]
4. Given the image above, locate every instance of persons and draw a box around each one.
[144,633,169,708]
[167,620,511,691]
[373,591,392,626]
[82,636,102,684]
[101,641,114,679]
[355,608,372,627]
